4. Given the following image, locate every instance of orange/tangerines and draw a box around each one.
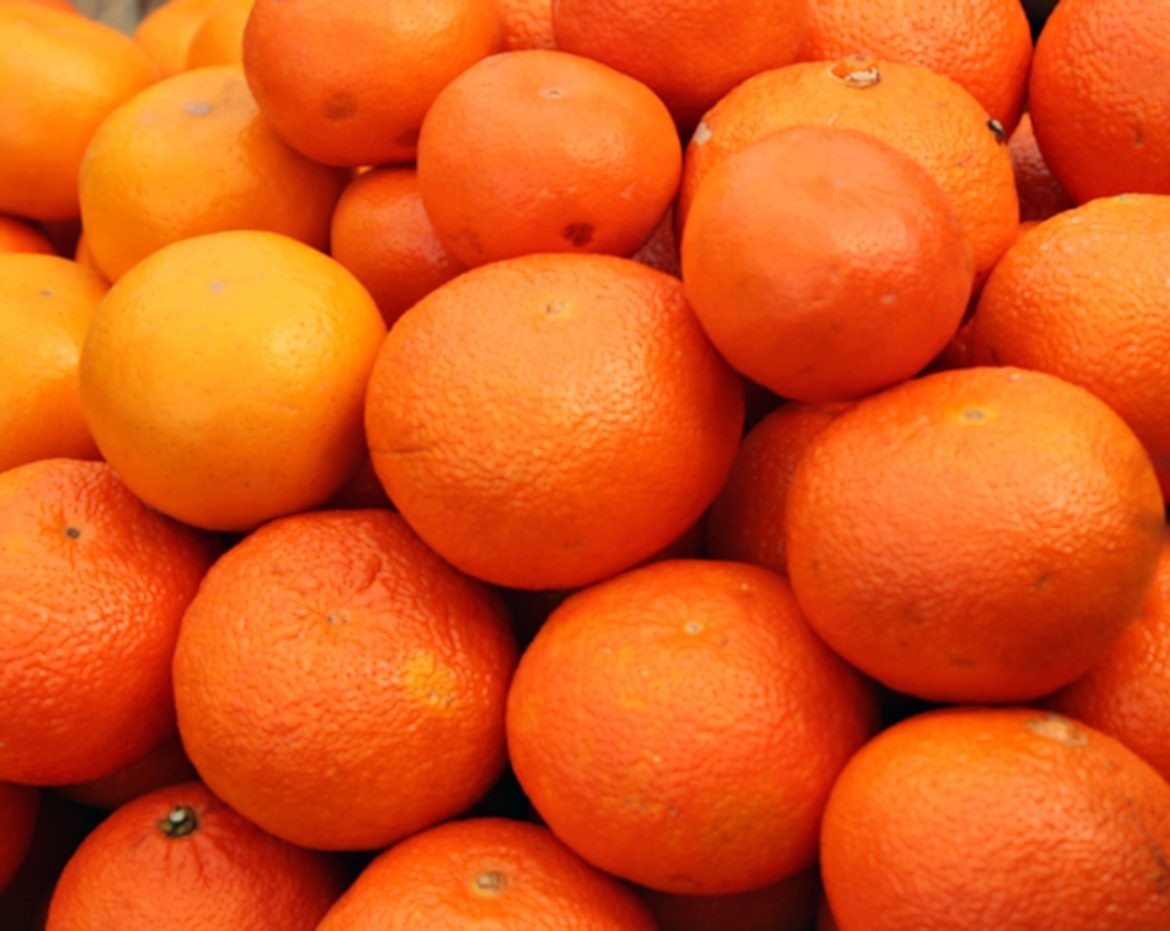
[0,0,1169,931]
